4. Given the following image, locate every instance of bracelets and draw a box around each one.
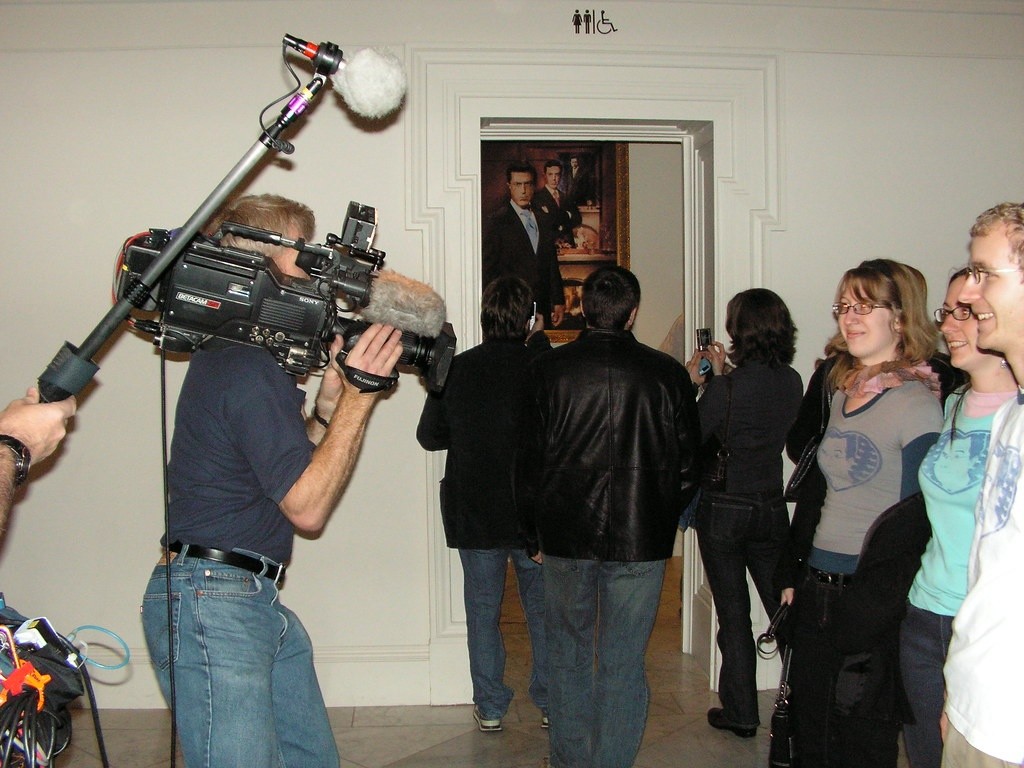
[311,408,329,430]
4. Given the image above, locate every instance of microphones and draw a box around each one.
[283,34,407,120]
[320,267,446,338]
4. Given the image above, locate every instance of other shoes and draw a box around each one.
[542,717,548,729]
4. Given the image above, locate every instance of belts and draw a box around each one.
[807,565,854,586]
[169,539,286,583]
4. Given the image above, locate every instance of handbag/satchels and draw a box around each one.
[691,375,732,495]
[783,359,831,503]
[756,604,793,768]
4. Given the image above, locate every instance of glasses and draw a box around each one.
[832,301,891,315]
[964,265,1024,285]
[933,305,971,323]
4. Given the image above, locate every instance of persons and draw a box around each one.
[766,200,1024,768]
[141,190,404,768]
[0,385,79,768]
[686,287,805,740]
[414,274,557,733]
[513,263,703,768]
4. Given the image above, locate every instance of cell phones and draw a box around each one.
[529,302,537,330]
[697,357,711,375]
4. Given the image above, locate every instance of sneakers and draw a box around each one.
[472,704,502,732]
[707,707,757,738]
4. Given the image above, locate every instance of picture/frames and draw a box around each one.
[553,149,599,212]
[517,141,616,263]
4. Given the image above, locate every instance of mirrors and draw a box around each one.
[480,140,633,345]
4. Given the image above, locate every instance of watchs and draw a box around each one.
[0,434,32,486]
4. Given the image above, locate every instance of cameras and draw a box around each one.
[697,327,712,351]
